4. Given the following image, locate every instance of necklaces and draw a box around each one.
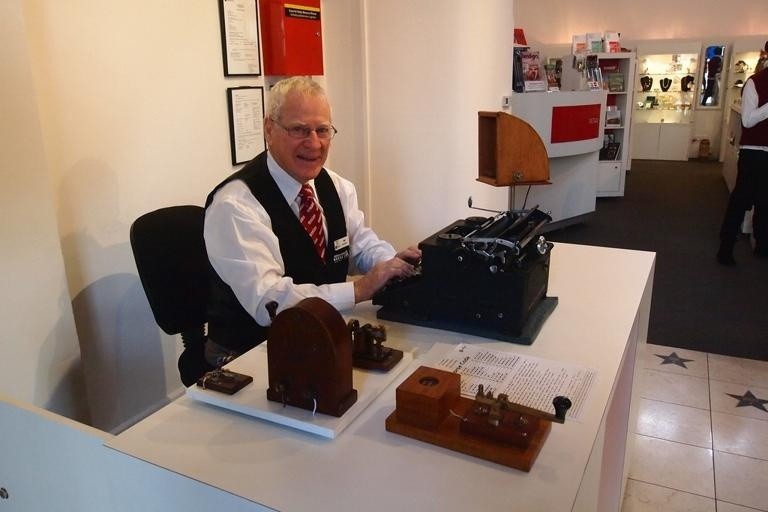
[644,79,651,89]
[663,79,670,89]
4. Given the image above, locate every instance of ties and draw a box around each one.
[297,182,328,262]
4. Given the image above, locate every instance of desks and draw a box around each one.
[108,243,656,512]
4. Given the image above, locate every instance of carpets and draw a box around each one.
[539,157,764,363]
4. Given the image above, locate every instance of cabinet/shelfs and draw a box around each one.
[597,50,744,197]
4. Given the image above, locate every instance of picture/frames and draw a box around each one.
[227,86,267,166]
[218,0,261,77]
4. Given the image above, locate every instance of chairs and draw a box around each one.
[130,206,232,388]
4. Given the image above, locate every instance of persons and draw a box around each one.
[717,40,768,265]
[197,75,423,381]
[702,47,723,106]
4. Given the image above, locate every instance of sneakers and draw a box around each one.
[716,242,767,268]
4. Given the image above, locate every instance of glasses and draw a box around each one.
[271,118,338,141]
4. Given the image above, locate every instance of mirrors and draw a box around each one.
[695,39,728,110]
